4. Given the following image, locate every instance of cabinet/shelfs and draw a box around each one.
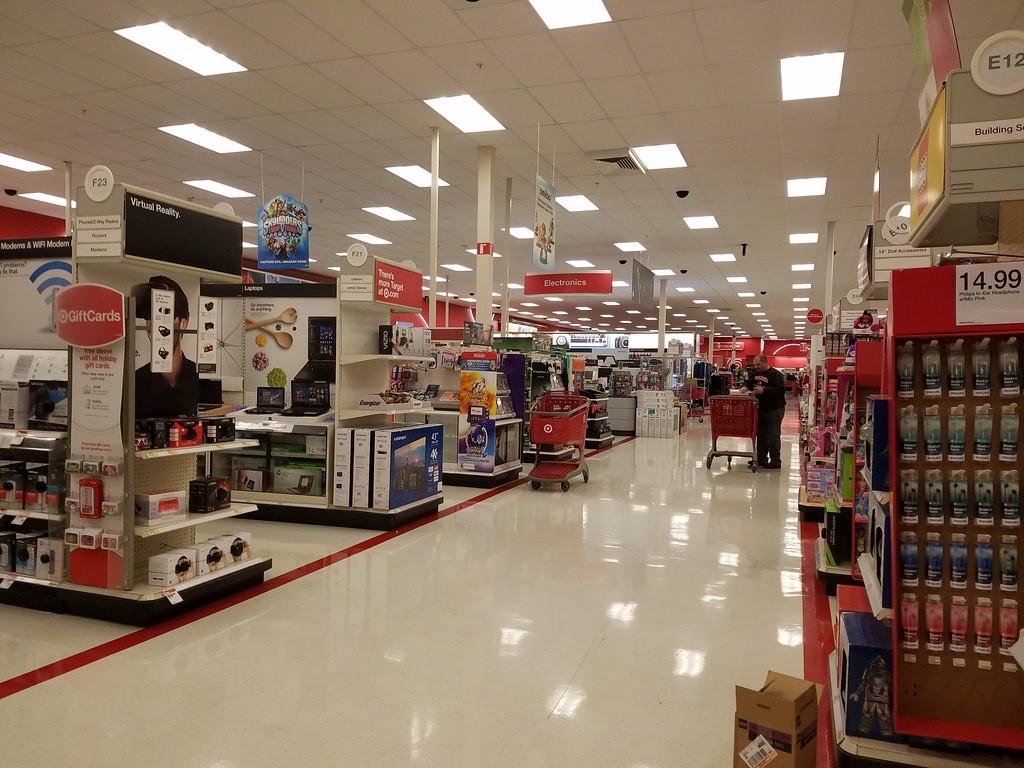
[0,427,272,629]
[522,397,615,464]
[424,338,524,490]
[799,333,892,762]
[228,276,443,531]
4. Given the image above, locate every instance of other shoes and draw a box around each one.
[747,458,768,466]
[764,460,781,469]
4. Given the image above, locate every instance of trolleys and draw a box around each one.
[706,394,758,473]
[684,384,708,423]
[525,391,592,491]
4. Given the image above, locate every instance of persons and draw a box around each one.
[129,275,198,419]
[857,475,867,495]
[740,354,787,469]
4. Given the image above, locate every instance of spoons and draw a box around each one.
[246,316,293,349]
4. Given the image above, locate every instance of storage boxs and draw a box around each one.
[379,325,431,357]
[733,670,825,768]
[274,466,322,497]
[805,480,837,494]
[0,460,66,516]
[841,446,853,499]
[1,529,69,583]
[231,457,268,493]
[823,501,852,570]
[271,434,327,455]
[807,468,836,482]
[148,531,251,587]
[133,490,187,527]
[189,477,232,514]
[636,390,688,439]
[133,416,235,452]
[833,583,972,753]
[807,491,835,504]
[333,423,443,511]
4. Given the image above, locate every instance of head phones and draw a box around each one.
[158,347,168,359]
[158,326,170,337]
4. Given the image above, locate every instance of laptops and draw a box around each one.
[281,380,331,416]
[287,475,314,495]
[246,387,285,414]
[294,316,337,383]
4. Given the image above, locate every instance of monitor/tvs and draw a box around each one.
[393,437,425,493]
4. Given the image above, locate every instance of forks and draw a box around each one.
[245,308,297,331]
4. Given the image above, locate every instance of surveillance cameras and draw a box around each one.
[676,190,689,199]
[834,251,836,255]
[308,227,313,232]
[761,292,766,295]
[680,269,687,273]
[619,260,627,264]
[730,325,734,327]
[4,189,17,196]
